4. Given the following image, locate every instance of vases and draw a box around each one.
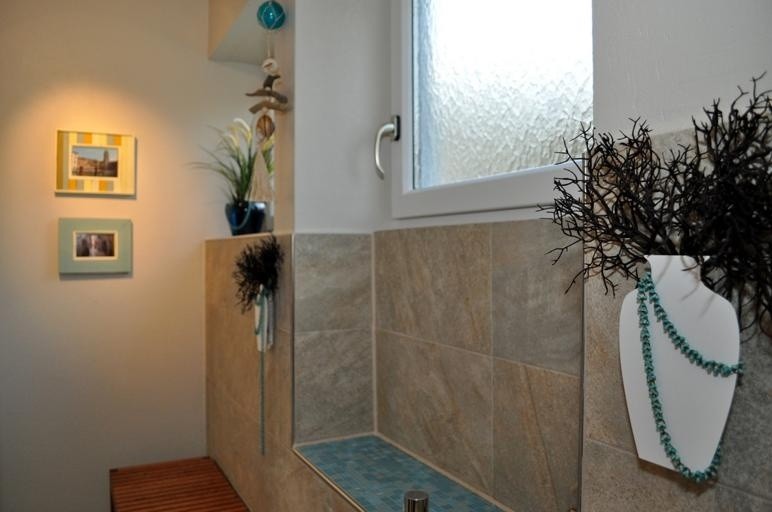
[619,255,742,478]
[254,292,275,350]
[225,200,268,235]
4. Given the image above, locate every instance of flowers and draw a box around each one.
[192,116,270,205]
[231,234,286,314]
[537,72,772,345]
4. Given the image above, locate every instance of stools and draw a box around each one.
[107,457,251,512]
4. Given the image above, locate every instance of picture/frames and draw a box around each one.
[54,130,137,197]
[58,218,134,277]
